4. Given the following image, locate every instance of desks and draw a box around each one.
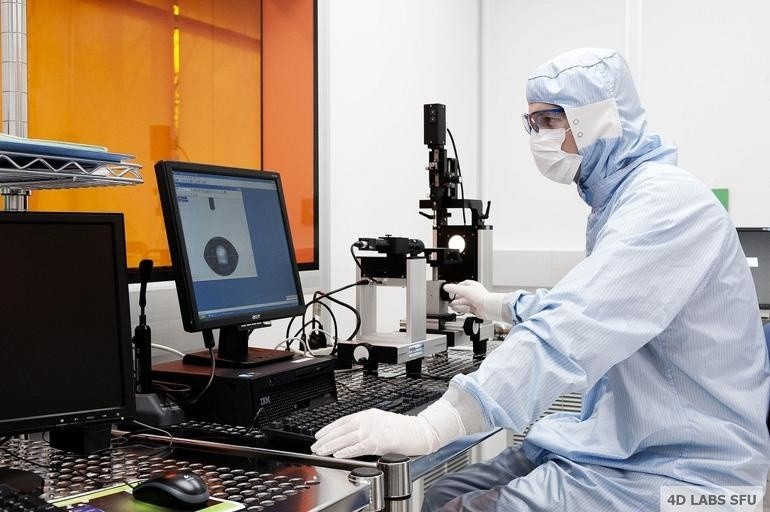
[107,342,505,512]
[1,427,385,512]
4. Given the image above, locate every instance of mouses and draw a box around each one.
[132,465,211,509]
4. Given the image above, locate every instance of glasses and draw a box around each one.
[521,108,569,136]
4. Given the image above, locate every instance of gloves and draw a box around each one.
[443,280,505,322]
[311,398,465,458]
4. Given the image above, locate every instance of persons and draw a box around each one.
[310,46,770,512]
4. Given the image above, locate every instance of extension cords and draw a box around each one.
[306,339,340,356]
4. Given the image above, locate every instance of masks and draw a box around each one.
[529,128,583,185]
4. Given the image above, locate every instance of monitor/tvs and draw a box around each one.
[153,158,307,369]
[0,210,137,433]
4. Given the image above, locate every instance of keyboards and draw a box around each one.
[264,380,445,442]
[0,490,62,511]
